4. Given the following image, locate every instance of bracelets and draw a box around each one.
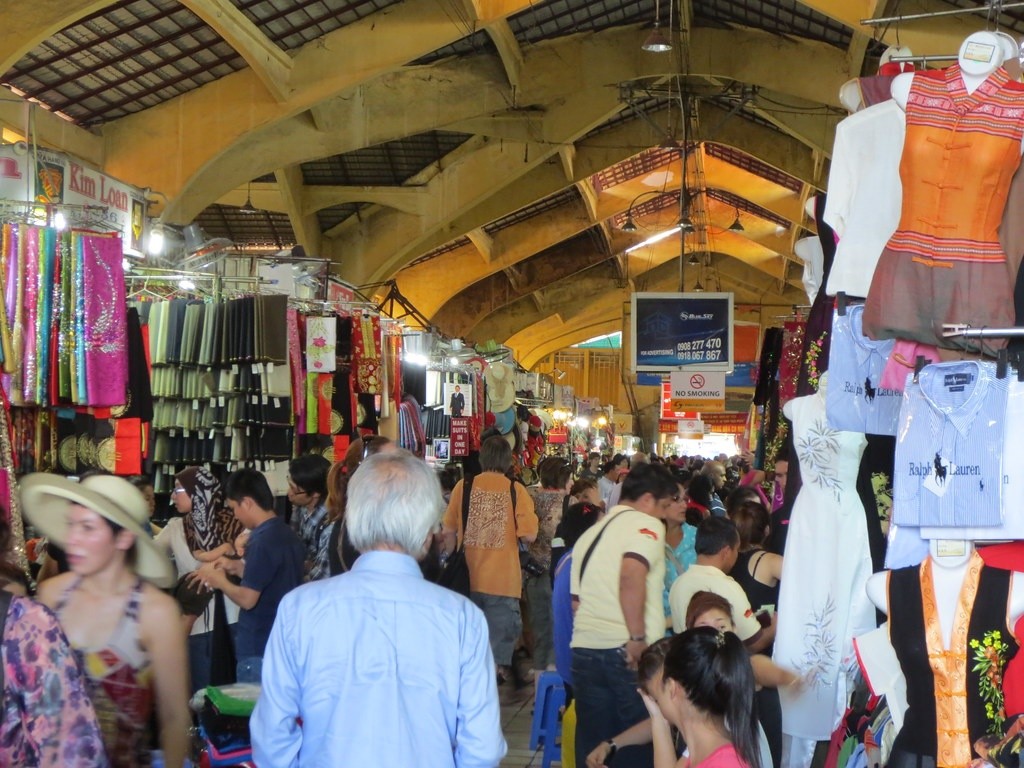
[607,739,616,753]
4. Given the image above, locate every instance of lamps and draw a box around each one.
[622,190,745,292]
[657,0,681,150]
[641,0,672,52]
[240,182,256,213]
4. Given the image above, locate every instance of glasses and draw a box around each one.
[286,476,308,496]
[671,495,691,504]
[173,487,187,495]
[561,460,570,468]
[362,434,374,459]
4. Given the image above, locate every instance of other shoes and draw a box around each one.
[497,668,506,687]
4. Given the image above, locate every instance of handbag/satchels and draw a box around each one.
[437,552,469,588]
[549,422,567,444]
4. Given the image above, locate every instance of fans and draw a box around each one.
[548,368,566,380]
[173,222,234,271]
[432,337,511,364]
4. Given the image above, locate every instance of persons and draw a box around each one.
[450,385,465,416]
[772,30,1024,768]
[0,434,801,768]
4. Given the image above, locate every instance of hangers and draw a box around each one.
[125,275,291,301]
[915,324,1024,381]
[824,291,866,316]
[0,200,124,238]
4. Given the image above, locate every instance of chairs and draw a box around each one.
[529,670,567,768]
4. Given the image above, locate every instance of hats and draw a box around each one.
[675,459,684,468]
[620,468,630,473]
[613,453,625,464]
[483,364,548,487]
[20,475,173,589]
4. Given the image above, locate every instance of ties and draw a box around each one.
[455,393,458,398]
